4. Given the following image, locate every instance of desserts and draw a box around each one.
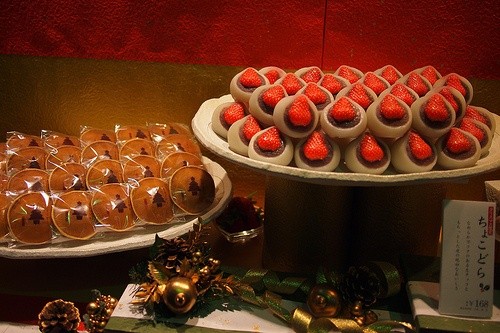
[212,65,496,174]
[0,123,215,244]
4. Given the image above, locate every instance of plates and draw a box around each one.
[0,156,231,259]
[191,93,500,186]
